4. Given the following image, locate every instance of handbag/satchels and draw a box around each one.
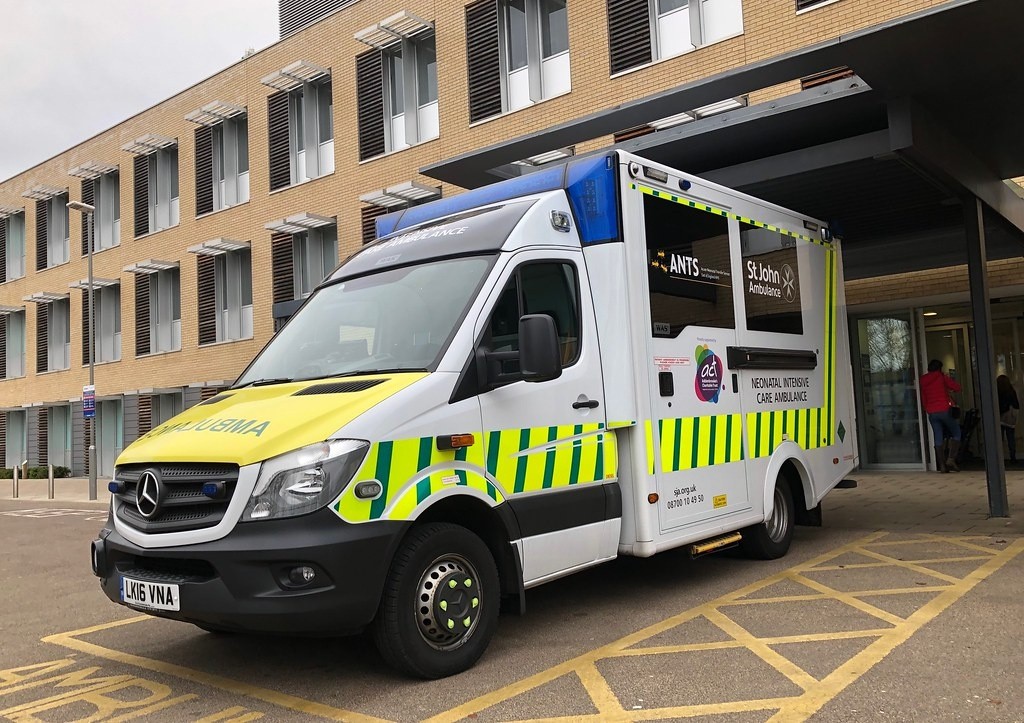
[949,407,962,420]
[1001,406,1019,429]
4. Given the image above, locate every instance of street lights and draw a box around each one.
[64,198,100,500]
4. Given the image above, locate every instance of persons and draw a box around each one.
[920,360,963,472]
[996,374,1020,463]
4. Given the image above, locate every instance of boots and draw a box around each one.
[934,444,949,473]
[946,439,960,472]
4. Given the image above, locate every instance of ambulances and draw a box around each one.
[90,147,862,680]
